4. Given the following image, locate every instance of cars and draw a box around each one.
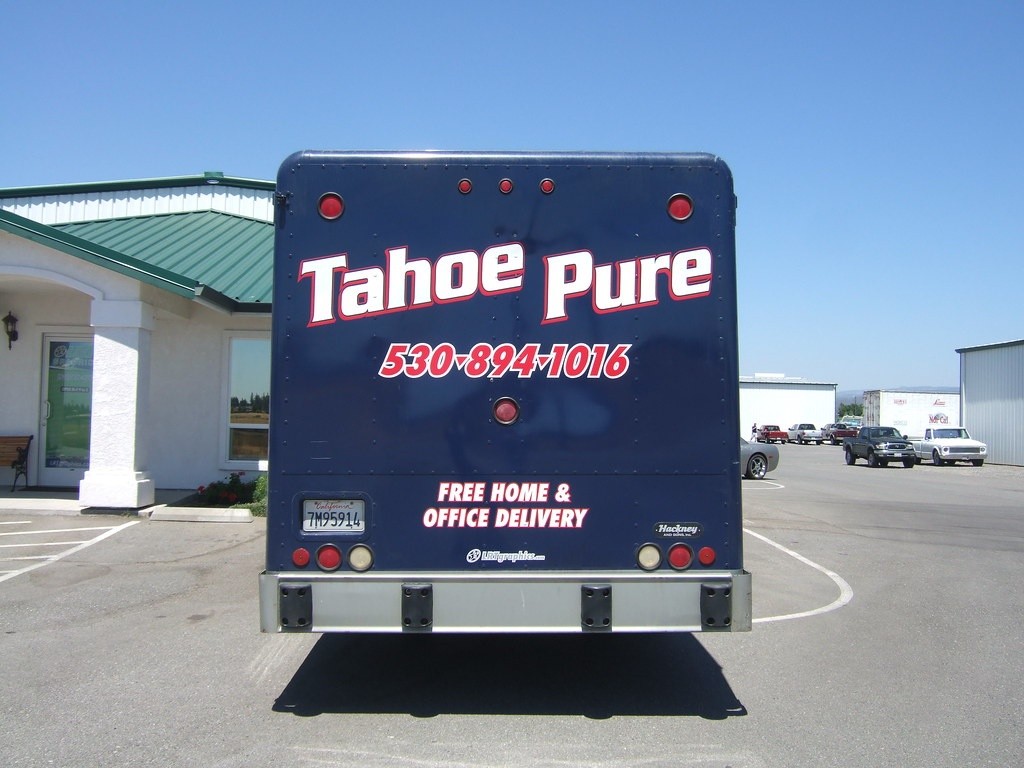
[841,415,863,427]
[740,436,780,479]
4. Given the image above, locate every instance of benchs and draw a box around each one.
[0,434,34,492]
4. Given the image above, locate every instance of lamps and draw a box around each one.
[1,311,18,350]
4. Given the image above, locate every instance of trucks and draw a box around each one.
[257,150,753,632]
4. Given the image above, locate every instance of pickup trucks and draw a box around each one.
[822,423,858,445]
[911,426,988,467]
[788,423,823,445]
[756,425,789,444]
[842,426,916,468]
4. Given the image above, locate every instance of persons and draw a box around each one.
[751,424,758,443]
